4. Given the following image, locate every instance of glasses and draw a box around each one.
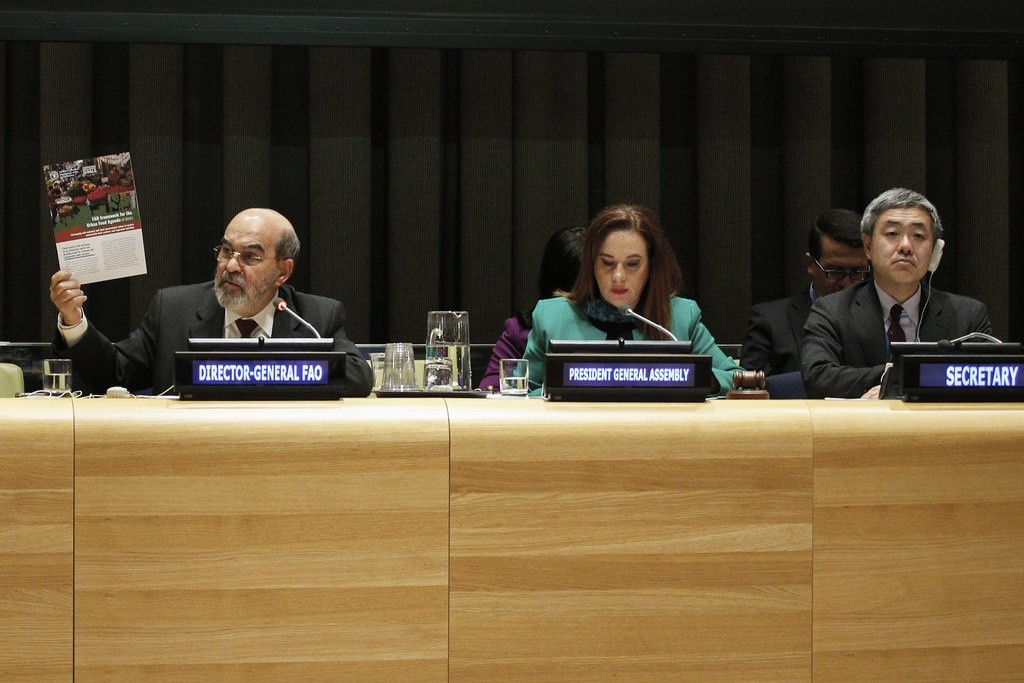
[812,255,872,285]
[213,245,293,266]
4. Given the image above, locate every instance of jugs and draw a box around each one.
[426,311,472,390]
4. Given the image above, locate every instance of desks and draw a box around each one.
[0,397,1024,683]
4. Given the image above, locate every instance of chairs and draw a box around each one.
[42,345,156,397]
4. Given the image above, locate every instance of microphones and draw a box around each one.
[273,297,322,339]
[936,332,1004,352]
[620,305,678,342]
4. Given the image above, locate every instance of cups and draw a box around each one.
[368,343,419,391]
[425,356,452,392]
[497,359,529,396]
[43,359,72,393]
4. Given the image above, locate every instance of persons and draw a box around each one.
[49,208,376,398]
[511,204,759,397]
[799,187,993,400]
[739,207,870,379]
[479,225,588,394]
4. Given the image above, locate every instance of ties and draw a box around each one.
[235,319,258,338]
[887,304,906,342]
